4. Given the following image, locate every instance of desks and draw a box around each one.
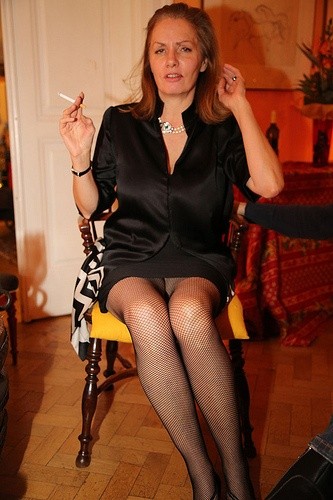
[232,161,333,338]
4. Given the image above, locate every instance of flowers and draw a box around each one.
[296,18,333,105]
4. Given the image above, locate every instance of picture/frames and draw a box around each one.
[194,0,326,89]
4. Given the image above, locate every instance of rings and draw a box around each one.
[231,76,237,81]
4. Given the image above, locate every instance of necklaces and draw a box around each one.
[157,113,186,134]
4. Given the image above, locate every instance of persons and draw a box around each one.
[58,3,286,500]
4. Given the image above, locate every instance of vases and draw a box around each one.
[299,104,333,168]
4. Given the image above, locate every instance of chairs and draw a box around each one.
[74,209,256,467]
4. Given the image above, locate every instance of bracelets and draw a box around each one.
[72,160,92,177]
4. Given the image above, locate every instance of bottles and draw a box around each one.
[265,111,279,156]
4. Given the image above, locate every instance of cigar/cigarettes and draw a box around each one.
[56,92,87,109]
